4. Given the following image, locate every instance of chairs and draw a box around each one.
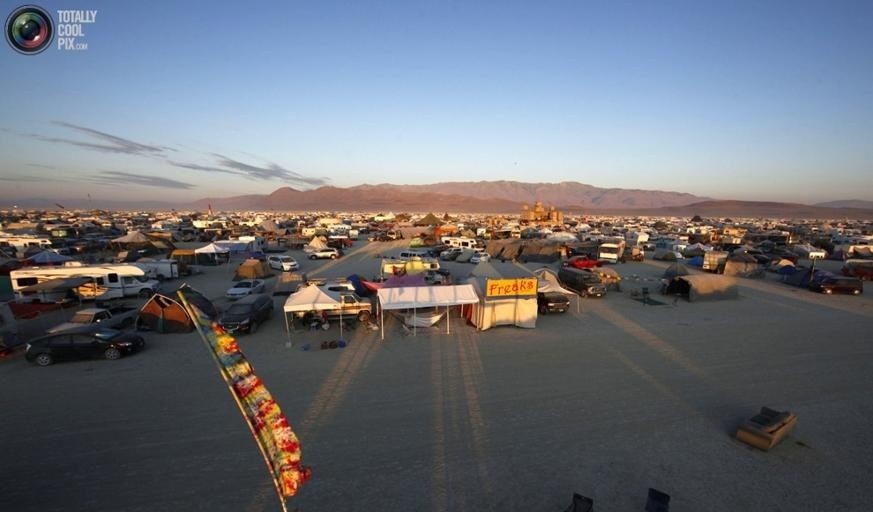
[562,493,593,512]
[643,487,669,512]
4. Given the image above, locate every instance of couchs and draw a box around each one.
[734,406,797,452]
